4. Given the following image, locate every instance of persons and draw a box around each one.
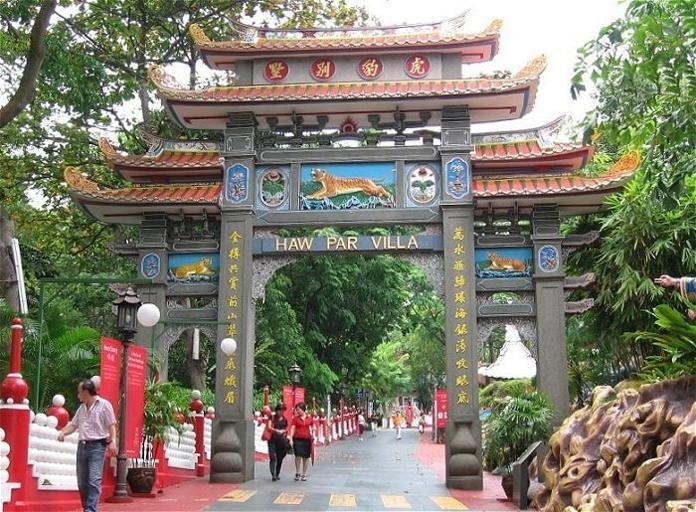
[267,403,292,481]
[394,410,403,439]
[289,402,315,481]
[419,412,424,434]
[58,378,118,511]
[357,407,368,441]
[370,409,380,438]
[654,275,696,304]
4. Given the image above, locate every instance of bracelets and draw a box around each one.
[109,439,115,445]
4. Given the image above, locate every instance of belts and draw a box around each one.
[78,438,107,444]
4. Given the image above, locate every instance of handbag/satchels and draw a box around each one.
[261,415,274,441]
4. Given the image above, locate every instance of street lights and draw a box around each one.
[287,361,302,407]
[112,284,141,496]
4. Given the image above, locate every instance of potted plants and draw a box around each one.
[119,383,194,494]
[478,382,553,500]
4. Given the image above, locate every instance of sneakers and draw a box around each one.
[294,473,306,480]
